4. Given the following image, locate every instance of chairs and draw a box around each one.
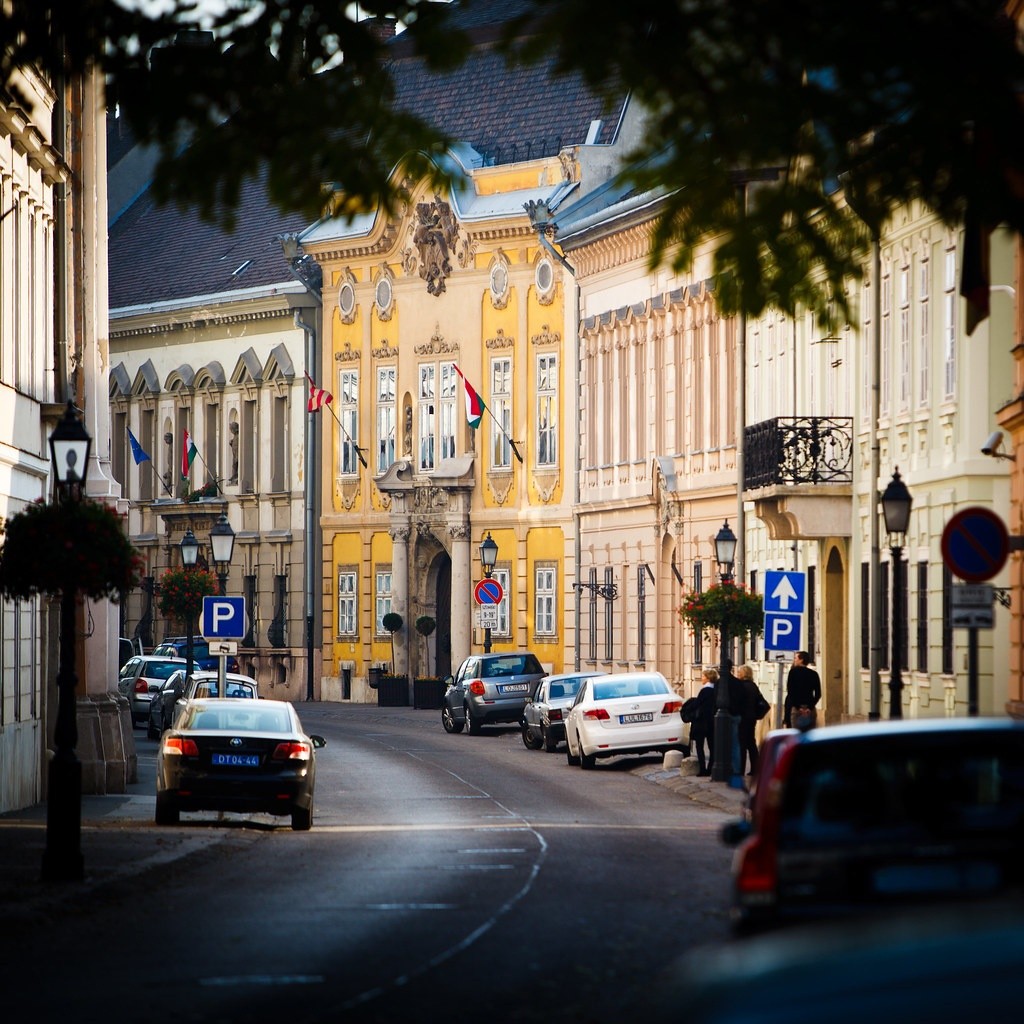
[199,713,218,730]
[637,681,654,696]
[231,690,246,697]
[550,686,565,698]
[512,665,525,674]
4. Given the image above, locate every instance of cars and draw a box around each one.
[563,670,692,769]
[520,672,606,753]
[718,716,1024,936]
[118,654,203,726]
[148,671,207,742]
[156,697,328,832]
[170,672,266,729]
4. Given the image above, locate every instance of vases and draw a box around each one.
[414,679,447,709]
[376,676,407,707]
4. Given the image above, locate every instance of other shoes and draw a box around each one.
[696,769,712,777]
[746,771,758,776]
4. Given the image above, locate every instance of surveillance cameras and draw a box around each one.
[981,432,1005,455]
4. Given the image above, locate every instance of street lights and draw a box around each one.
[208,509,237,596]
[178,526,204,684]
[881,467,913,722]
[710,516,738,781]
[41,397,92,891]
[476,532,499,654]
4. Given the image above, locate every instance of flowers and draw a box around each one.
[0,495,145,605]
[383,671,402,678]
[679,579,763,646]
[155,565,222,625]
[416,675,439,680]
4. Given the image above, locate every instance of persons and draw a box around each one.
[229,422,239,479]
[163,432,173,494]
[736,665,761,776]
[404,406,412,455]
[783,651,822,731]
[690,669,719,777]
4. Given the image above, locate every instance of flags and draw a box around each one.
[128,431,151,465]
[454,365,486,429]
[306,373,333,412]
[181,430,198,481]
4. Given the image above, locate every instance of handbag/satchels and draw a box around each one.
[791,705,815,729]
[753,696,773,721]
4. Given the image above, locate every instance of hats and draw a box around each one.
[729,664,755,682]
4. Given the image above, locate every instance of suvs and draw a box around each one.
[442,651,548,736]
[151,636,240,675]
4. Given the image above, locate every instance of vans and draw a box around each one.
[119,637,135,673]
